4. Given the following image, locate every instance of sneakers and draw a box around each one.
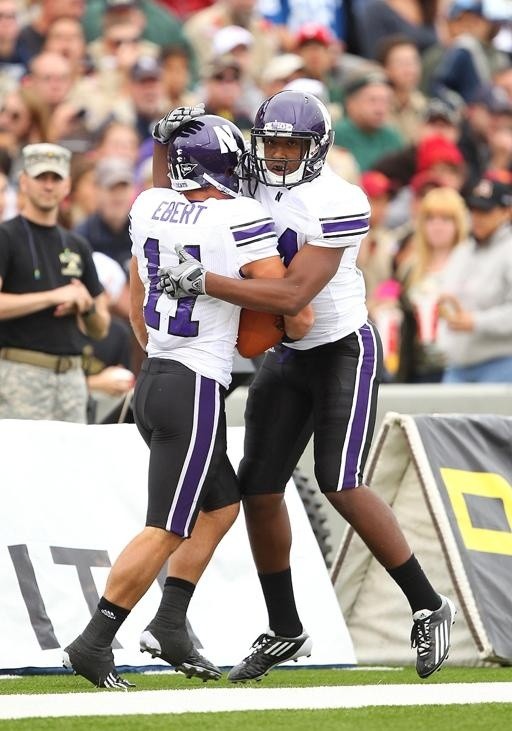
[138,622,222,681]
[411,594,458,678]
[60,638,137,689]
[227,630,314,684]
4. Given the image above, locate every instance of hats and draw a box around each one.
[416,135,461,174]
[468,169,512,211]
[211,26,253,54]
[93,159,138,192]
[23,143,71,179]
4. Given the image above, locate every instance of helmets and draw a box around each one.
[256,94,334,186]
[166,114,247,198]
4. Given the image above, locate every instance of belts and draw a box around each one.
[1,348,86,369]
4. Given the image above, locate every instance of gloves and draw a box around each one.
[153,103,205,143]
[154,246,206,300]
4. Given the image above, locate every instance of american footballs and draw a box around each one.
[237,309,285,358]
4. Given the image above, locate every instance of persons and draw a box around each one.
[145,88,459,685]
[0,1,512,420]
[59,109,317,701]
